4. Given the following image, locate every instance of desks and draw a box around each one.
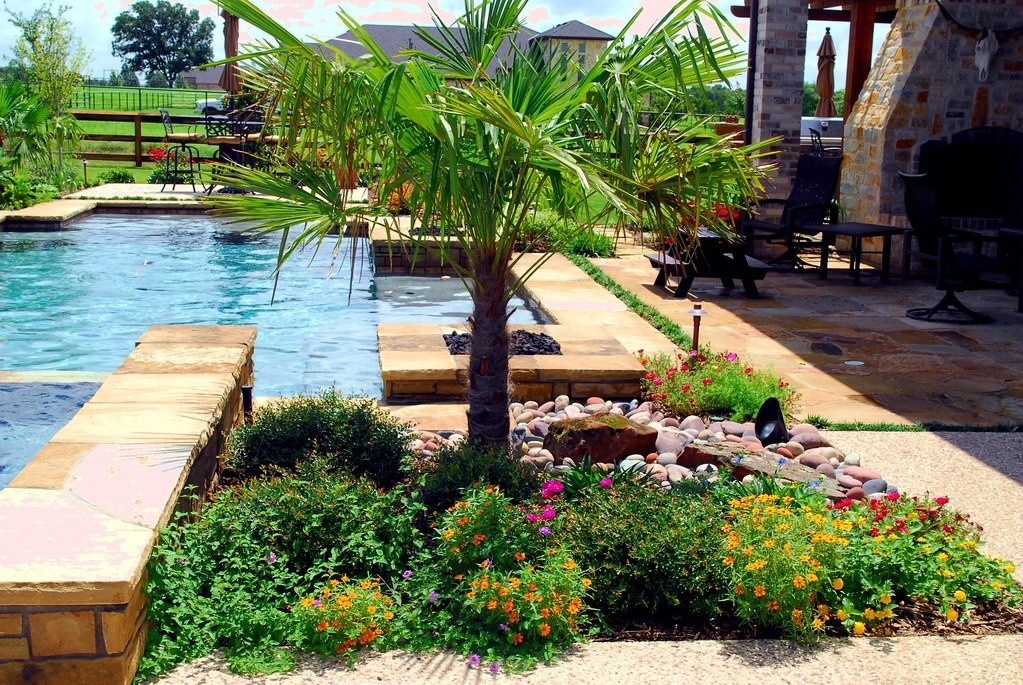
[196,120,265,196]
[801,222,912,286]
[654,227,761,300]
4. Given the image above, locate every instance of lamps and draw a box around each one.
[685,304,709,316]
[755,397,793,447]
[82,159,90,164]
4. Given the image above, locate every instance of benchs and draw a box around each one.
[643,252,689,266]
[723,250,773,280]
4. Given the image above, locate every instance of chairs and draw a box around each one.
[808,127,841,158]
[743,153,844,280]
[157,108,298,193]
[897,169,996,324]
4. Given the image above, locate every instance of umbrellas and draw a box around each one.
[815,26,839,118]
[217,6,245,135]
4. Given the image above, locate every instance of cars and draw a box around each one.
[193,92,282,116]
